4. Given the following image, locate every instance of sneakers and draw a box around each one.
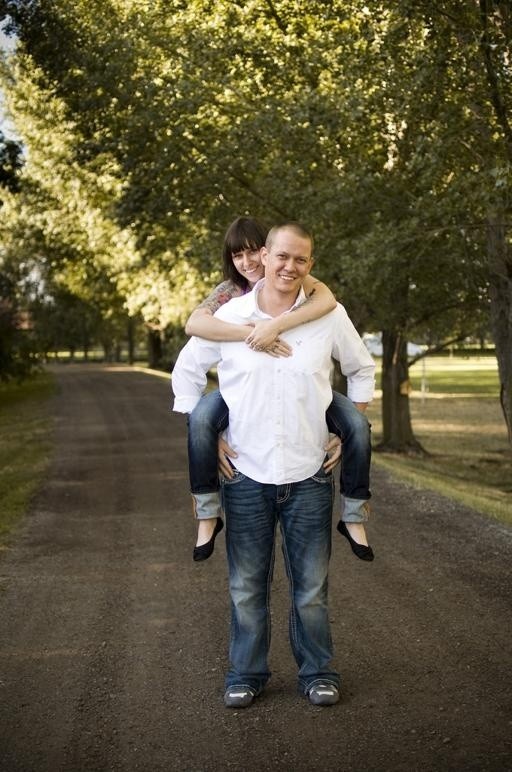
[223,684,261,707]
[304,675,341,705]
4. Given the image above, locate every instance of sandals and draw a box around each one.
[193,517,224,561]
[337,519,374,562]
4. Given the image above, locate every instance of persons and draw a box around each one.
[171,220,378,710]
[178,213,377,563]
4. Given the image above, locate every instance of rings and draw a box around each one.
[250,341,256,347]
[259,346,265,351]
[270,347,276,352]
[276,337,280,342]
[255,343,261,350]
[265,349,269,353]
[246,336,253,342]
[275,344,279,348]
